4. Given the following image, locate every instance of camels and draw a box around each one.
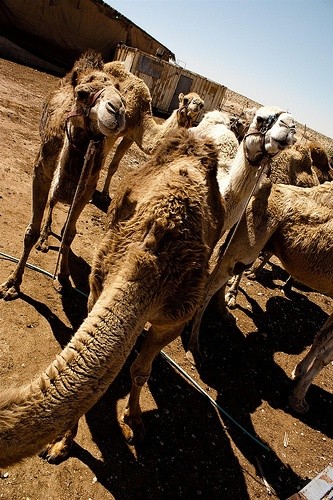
[0,52,332,473]
